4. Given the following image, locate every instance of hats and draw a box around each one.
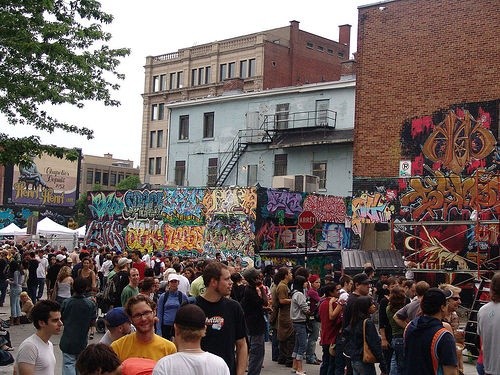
[118,257,132,267]
[156,253,162,257]
[423,288,454,308]
[55,254,66,262]
[352,273,372,283]
[309,274,319,282]
[105,307,131,328]
[67,257,72,262]
[174,304,206,328]
[242,267,262,278]
[168,274,179,282]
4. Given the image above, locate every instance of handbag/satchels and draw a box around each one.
[362,318,384,364]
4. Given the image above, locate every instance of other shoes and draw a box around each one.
[90,333,94,339]
[296,371,306,375]
[317,359,322,362]
[3,346,14,351]
[291,368,306,373]
[313,361,320,365]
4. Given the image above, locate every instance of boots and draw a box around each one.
[8,317,13,325]
[14,317,20,325]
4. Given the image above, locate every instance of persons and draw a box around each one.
[378,271,462,375]
[475,273,500,375]
[0,239,382,375]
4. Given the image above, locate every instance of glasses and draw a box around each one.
[451,296,462,301]
[315,282,321,283]
[132,310,153,319]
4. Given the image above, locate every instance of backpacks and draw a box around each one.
[153,259,162,276]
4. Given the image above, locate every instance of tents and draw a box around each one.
[0,217,86,252]
